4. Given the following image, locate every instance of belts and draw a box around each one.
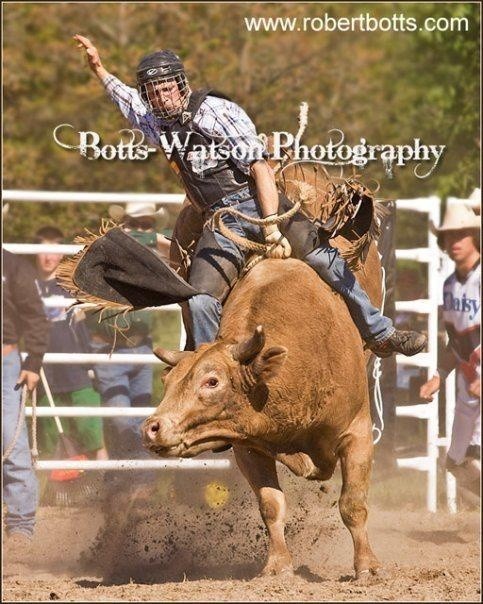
[3,343,17,355]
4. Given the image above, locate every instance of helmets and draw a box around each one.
[134,50,192,124]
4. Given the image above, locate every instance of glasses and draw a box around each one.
[128,219,153,230]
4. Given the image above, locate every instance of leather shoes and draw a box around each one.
[370,329,427,358]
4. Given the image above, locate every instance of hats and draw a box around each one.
[426,203,480,237]
[108,201,168,230]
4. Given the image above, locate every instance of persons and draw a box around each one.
[2,196,480,550]
[71,34,428,367]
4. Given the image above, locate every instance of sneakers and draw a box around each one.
[5,512,35,543]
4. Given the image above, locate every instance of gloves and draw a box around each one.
[260,212,291,259]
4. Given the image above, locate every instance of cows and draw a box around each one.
[137,131,387,586]
